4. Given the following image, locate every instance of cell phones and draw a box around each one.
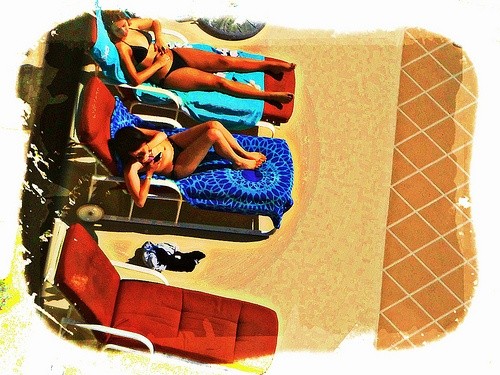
[154,152,162,163]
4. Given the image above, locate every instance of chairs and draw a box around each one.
[31,218,278,375]
[67,80,296,241]
[85,10,296,123]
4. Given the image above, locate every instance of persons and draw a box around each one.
[114,121,267,208]
[101,10,296,104]
